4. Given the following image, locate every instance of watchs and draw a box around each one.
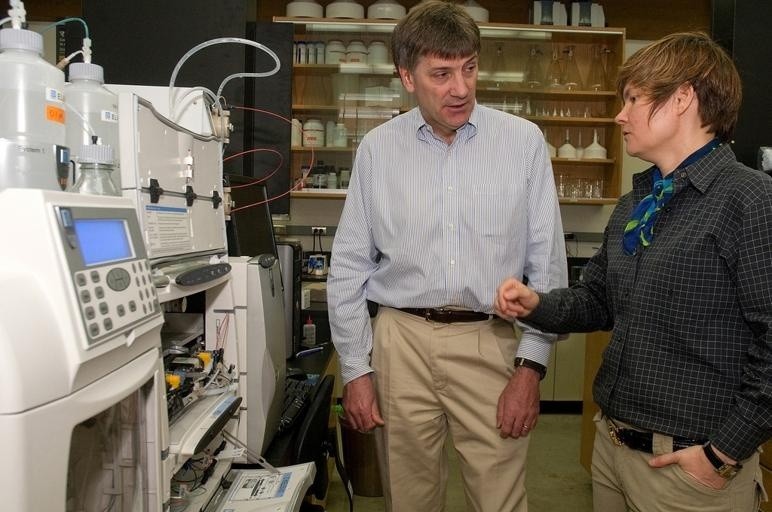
[699,436,744,485]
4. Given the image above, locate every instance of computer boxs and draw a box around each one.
[275,242,302,359]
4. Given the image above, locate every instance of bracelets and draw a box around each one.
[511,354,550,382]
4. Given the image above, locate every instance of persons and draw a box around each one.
[492,32,770,511]
[325,1,573,510]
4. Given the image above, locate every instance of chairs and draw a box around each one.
[292,375,354,512]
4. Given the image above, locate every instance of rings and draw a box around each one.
[522,423,531,432]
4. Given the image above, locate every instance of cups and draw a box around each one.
[554,174,609,198]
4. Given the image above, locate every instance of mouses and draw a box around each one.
[288,366,308,381]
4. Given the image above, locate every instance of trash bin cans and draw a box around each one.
[337,410,384,497]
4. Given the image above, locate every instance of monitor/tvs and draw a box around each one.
[224,174,278,263]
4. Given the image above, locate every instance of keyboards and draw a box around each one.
[278,376,314,429]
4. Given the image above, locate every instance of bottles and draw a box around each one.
[293,38,388,64]
[293,157,352,193]
[492,40,618,90]
[302,117,324,147]
[325,120,348,148]
[0,0,123,196]
[302,315,316,345]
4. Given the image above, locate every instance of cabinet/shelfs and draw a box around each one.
[273,18,627,205]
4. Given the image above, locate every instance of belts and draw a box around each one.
[603,417,702,454]
[398,307,496,324]
